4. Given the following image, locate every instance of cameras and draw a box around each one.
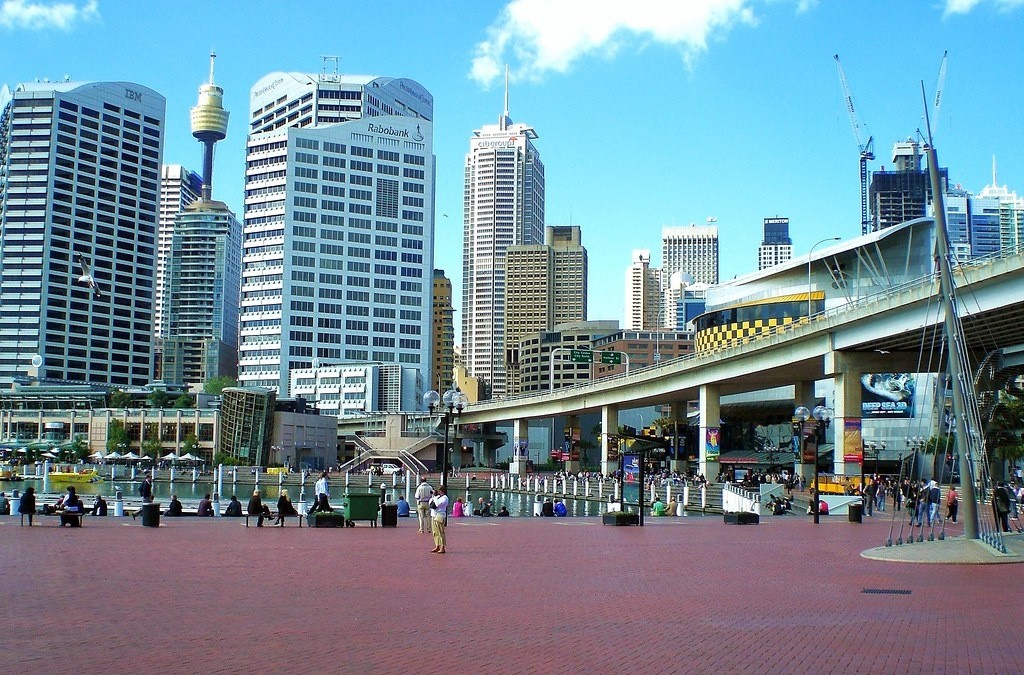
[430,490,438,496]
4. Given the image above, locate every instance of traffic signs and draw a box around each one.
[601,351,622,365]
[570,350,594,363]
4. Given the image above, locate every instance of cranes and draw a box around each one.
[832,52,877,237]
[931,51,949,150]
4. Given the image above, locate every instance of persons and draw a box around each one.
[974,467,1024,532]
[226,489,296,528]
[0,492,9,514]
[131,475,153,520]
[644,468,806,516]
[398,476,464,552]
[449,469,548,486]
[289,463,405,482]
[310,473,331,511]
[18,487,107,526]
[554,468,621,484]
[841,473,959,526]
[227,465,256,478]
[540,498,567,516]
[198,494,214,516]
[164,496,182,516]
[9,456,216,476]
[473,497,509,518]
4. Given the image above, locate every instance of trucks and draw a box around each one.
[365,463,403,476]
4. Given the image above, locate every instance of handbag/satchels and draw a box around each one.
[434,513,444,523]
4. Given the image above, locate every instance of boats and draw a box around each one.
[47,464,107,485]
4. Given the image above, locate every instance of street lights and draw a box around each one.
[423,389,470,527]
[271,445,281,468]
[191,443,202,471]
[656,307,670,369]
[904,434,925,482]
[427,368,442,410]
[808,238,842,325]
[500,359,512,402]
[795,405,834,524]
[870,440,887,476]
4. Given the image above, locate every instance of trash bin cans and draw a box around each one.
[342,492,381,528]
[848,503,862,523]
[381,504,398,527]
[141,503,159,527]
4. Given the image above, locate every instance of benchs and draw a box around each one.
[245,514,303,528]
[19,510,83,528]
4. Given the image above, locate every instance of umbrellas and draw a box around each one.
[89,451,202,461]
[17,448,76,458]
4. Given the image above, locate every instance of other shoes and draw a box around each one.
[915,523,921,526]
[438,550,445,553]
[257,524,263,527]
[428,531,431,533]
[952,522,958,524]
[268,517,275,520]
[281,525,283,527]
[275,521,278,525]
[418,531,424,533]
[430,549,439,552]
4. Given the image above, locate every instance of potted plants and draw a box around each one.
[602,511,639,528]
[307,510,344,528]
[723,511,760,525]
